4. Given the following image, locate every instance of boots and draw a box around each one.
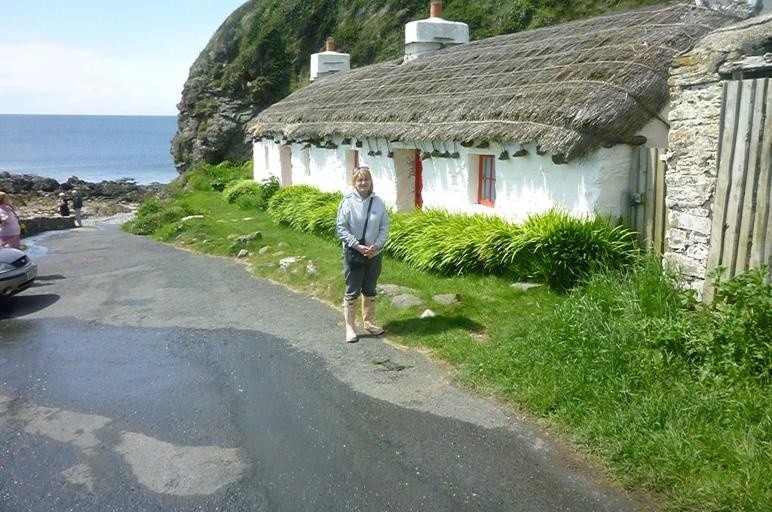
[360,293,386,336]
[342,296,360,343]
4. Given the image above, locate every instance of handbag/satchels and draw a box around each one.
[342,239,369,272]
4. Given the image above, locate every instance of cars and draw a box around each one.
[0,246,37,300]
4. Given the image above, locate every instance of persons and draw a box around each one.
[0,191,20,249]
[71,190,83,227]
[336,166,388,343]
[58,193,69,216]
